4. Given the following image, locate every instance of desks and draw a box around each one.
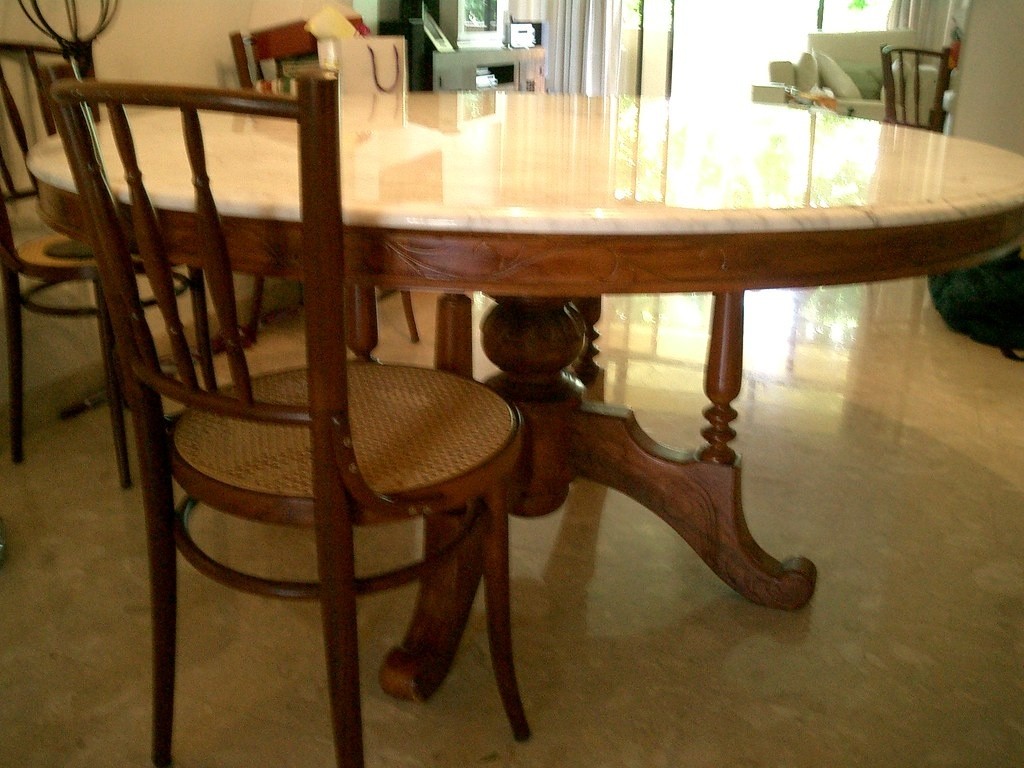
[23,88,1024,708]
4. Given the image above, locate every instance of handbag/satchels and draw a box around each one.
[927,249,1024,363]
[317,33,406,95]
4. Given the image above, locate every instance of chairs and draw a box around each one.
[0,40,218,490]
[229,16,421,358]
[880,43,952,132]
[40,60,531,768]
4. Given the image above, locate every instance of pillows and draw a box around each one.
[881,59,909,103]
[795,52,819,93]
[812,47,862,100]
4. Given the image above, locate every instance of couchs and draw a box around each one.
[751,28,940,128]
